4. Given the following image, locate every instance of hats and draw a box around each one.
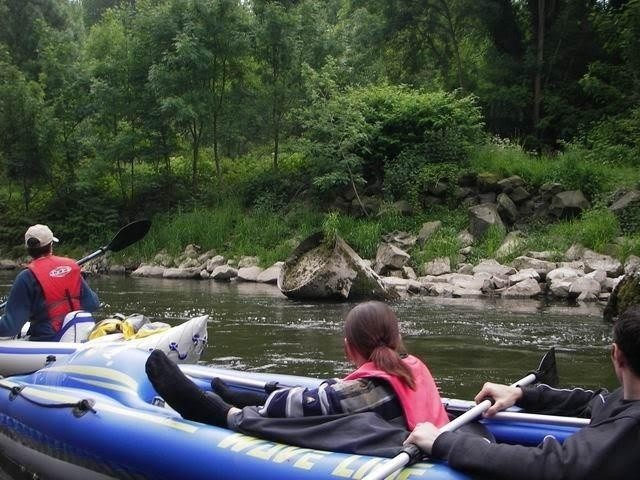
[24,223,60,249]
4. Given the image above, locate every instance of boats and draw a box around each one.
[0,344,593,480]
[0,311,211,378]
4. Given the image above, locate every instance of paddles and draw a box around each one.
[361,347,560,480]
[1,219,154,310]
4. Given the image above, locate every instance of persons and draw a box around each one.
[259,301,449,437]
[0,222,101,341]
[146,305,638,479]
